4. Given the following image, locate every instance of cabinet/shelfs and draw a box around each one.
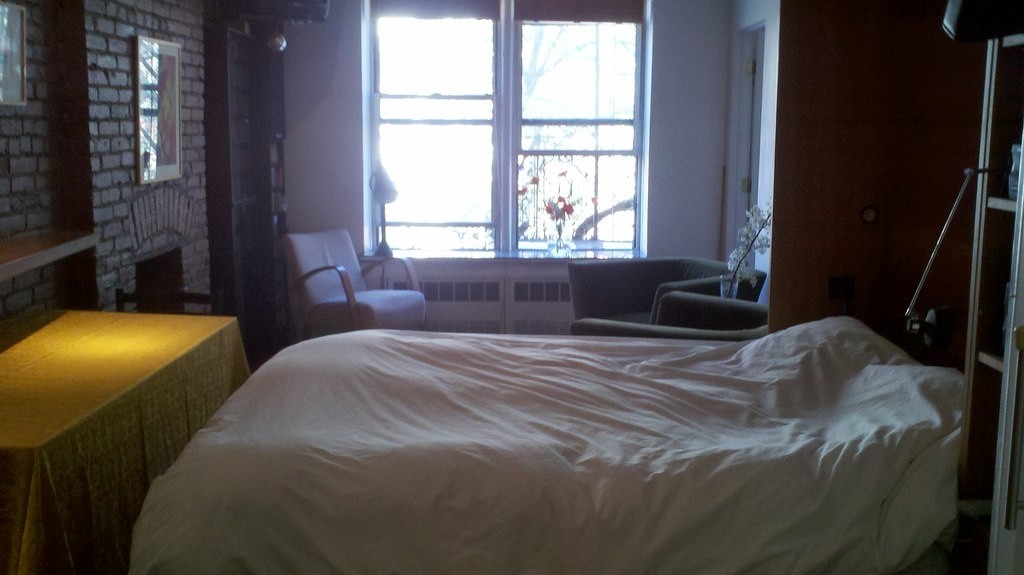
[952,32,1024,504]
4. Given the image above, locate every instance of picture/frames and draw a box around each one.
[135,33,184,185]
[0,0,26,106]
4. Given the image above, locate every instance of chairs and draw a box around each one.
[567,256,769,341]
[280,229,425,347]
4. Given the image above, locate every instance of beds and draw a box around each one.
[126,315,964,575]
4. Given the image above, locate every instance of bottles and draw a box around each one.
[1009,144,1021,200]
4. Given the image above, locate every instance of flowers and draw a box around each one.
[727,204,772,288]
[545,195,576,244]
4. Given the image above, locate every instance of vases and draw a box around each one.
[720,274,738,299]
[547,237,573,259]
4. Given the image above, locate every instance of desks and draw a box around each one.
[0,306,253,575]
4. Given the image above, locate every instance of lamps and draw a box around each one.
[369,169,398,259]
[237,0,331,51]
